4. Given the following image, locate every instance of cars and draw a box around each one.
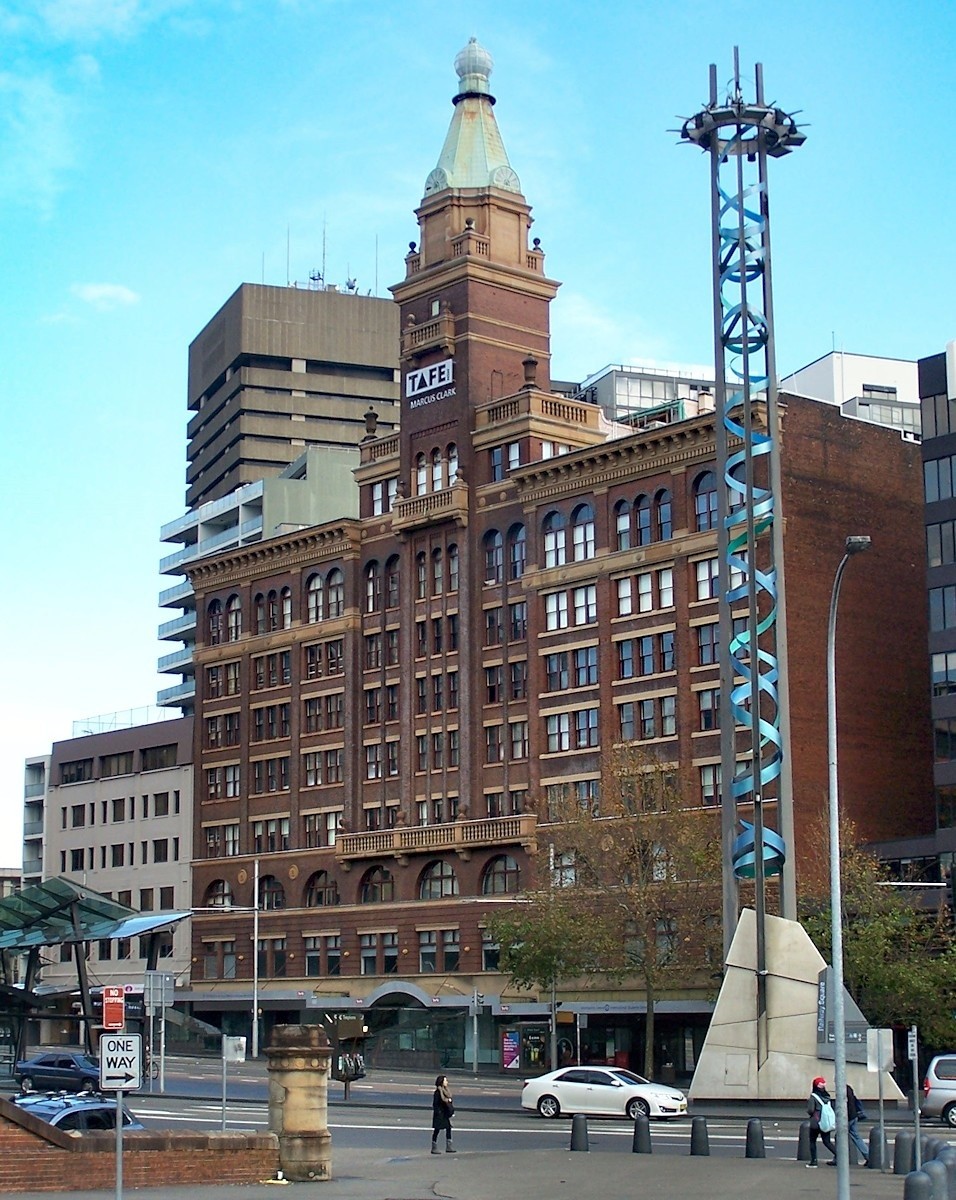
[520,1065,688,1121]
[14,1051,130,1098]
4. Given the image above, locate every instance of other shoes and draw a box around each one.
[864,1160,870,1166]
[809,1093,838,1132]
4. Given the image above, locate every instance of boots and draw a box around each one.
[432,1142,442,1154]
[446,1139,457,1153]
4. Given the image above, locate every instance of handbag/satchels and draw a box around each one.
[436,1090,455,1119]
[853,1094,868,1122]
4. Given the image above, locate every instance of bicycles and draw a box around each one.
[145,1060,160,1079]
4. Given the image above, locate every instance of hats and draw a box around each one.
[813,1077,826,1089]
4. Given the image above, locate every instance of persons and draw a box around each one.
[806,1076,835,1168]
[431,1076,456,1155]
[557,1043,589,1070]
[825,1084,869,1166]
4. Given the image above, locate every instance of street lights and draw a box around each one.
[825,535,873,1200]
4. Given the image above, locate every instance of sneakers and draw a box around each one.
[826,1161,838,1166]
[806,1158,819,1169]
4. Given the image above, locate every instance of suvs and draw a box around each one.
[25,1096,144,1133]
[920,1054,956,1127]
